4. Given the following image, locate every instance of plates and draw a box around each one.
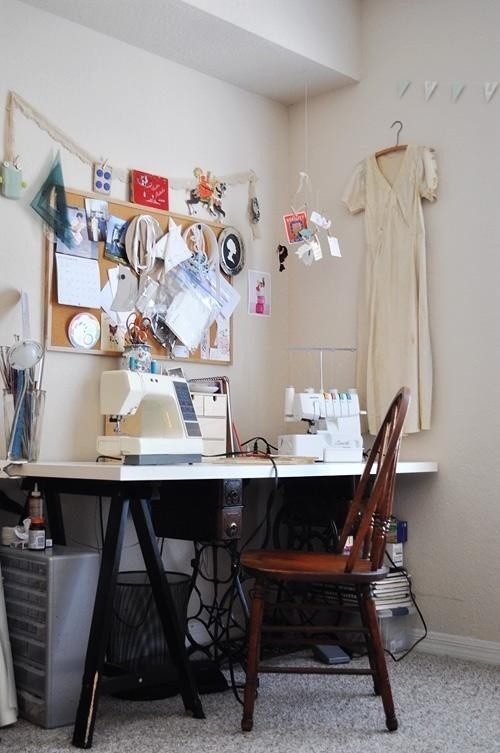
[191,385,218,393]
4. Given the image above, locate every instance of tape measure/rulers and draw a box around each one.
[30,148,76,250]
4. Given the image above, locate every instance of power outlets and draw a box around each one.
[222,479,243,506]
[222,507,243,540]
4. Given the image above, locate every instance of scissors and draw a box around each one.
[125,312,152,344]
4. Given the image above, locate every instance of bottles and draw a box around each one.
[28,516,47,551]
[28,483,44,520]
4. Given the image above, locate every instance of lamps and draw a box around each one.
[3,340,40,479]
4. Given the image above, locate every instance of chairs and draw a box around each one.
[238,387,410,733]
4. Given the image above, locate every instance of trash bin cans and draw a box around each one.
[106,570,193,701]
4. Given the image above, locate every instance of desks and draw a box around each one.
[0,458,439,748]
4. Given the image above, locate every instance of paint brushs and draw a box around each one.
[0,333,37,462]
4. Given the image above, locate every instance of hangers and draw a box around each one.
[376,121,407,157]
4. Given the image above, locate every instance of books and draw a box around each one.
[304,568,414,611]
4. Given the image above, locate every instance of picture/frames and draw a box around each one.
[46,184,236,367]
